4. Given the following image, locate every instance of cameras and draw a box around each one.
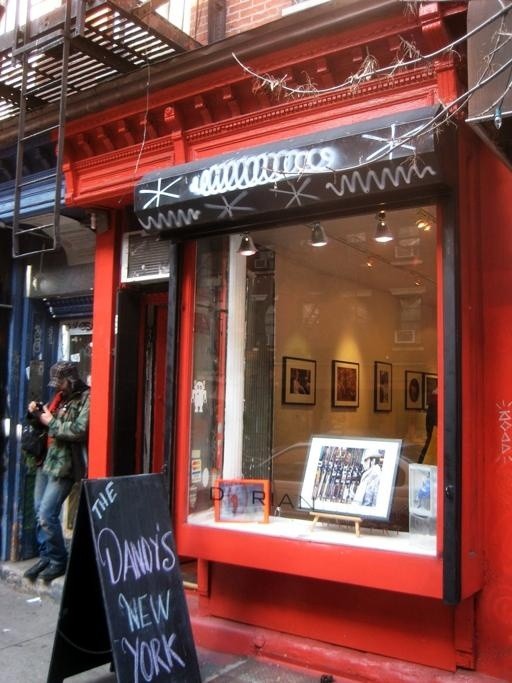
[35,402,44,413]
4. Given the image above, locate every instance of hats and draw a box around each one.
[48,361,80,388]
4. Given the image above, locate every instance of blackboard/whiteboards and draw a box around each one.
[84,471,202,683]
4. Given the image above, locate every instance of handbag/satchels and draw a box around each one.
[23,413,47,459]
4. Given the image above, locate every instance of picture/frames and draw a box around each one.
[281,356,316,406]
[376,361,392,412]
[213,479,271,524]
[424,372,438,411]
[409,462,438,521]
[405,370,423,410]
[332,360,359,407]
[295,434,404,523]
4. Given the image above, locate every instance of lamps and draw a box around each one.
[373,201,393,244]
[308,216,328,248]
[234,228,257,257]
[493,106,502,129]
[414,206,437,232]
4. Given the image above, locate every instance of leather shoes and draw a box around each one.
[24,559,49,577]
[38,560,67,579]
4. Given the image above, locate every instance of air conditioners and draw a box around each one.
[395,246,413,259]
[394,330,415,344]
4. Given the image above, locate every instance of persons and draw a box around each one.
[23,361,92,582]
[353,448,382,506]
